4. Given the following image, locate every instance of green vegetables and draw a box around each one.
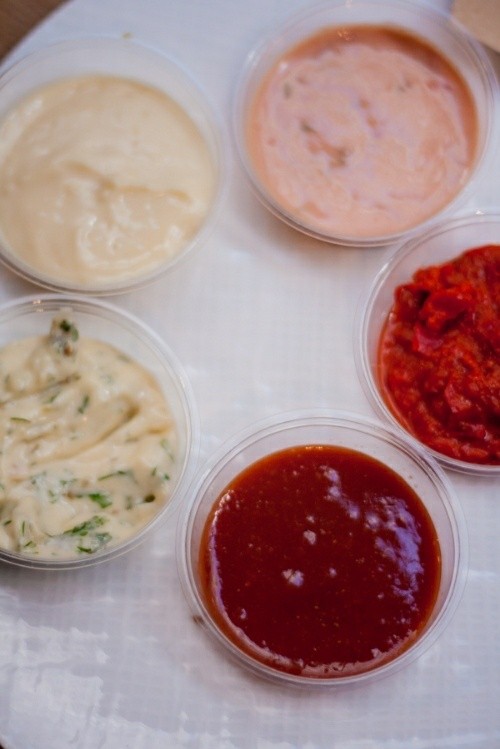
[0,319,176,554]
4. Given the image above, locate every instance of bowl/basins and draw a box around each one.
[173,406,470,693]
[352,208,500,480]
[0,34,228,298]
[0,295,202,572]
[229,1,500,249]
